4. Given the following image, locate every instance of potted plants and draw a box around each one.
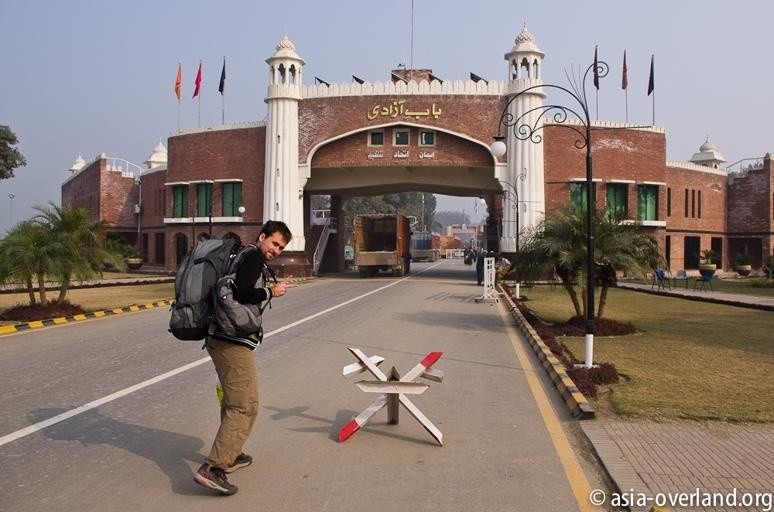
[691,248,721,276]
[124,244,146,269]
[732,252,755,276]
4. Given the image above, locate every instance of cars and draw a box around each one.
[344,243,353,269]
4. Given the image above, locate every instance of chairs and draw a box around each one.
[648,267,714,294]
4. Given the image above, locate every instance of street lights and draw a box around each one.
[208,200,246,239]
[474,169,527,301]
[491,59,610,371]
[7,192,15,231]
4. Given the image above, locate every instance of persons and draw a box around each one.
[476,249,488,286]
[486,250,498,288]
[463,246,483,273]
[192,220,297,493]
[497,255,512,266]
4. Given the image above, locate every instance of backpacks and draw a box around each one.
[165,236,242,343]
[210,273,266,341]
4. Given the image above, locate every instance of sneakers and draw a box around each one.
[223,451,254,476]
[189,461,240,497]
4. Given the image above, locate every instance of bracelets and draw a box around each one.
[270,287,275,297]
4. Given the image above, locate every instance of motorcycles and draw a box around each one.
[464,246,477,265]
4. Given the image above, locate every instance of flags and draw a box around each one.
[646,55,656,96]
[192,64,201,99]
[172,64,183,102]
[622,53,628,89]
[593,47,600,90]
[216,60,227,95]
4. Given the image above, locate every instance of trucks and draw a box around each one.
[410,231,441,263]
[352,214,417,275]
